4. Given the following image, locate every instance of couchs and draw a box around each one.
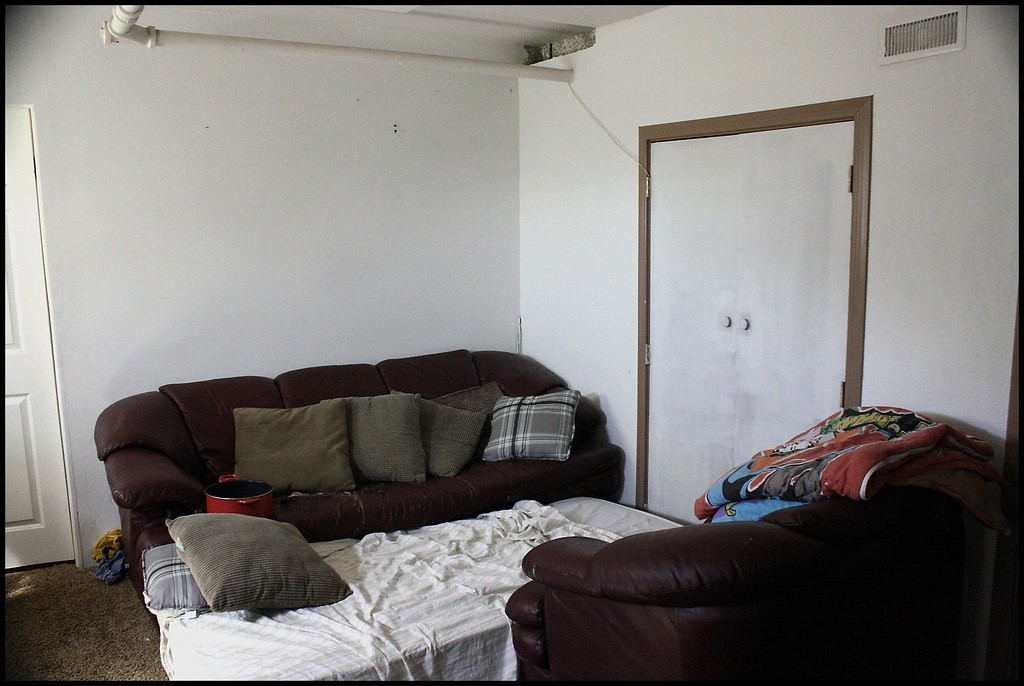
[503,498,986,685]
[95,348,628,612]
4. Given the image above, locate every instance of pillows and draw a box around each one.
[389,389,487,479]
[166,514,352,613]
[427,380,505,430]
[141,542,216,612]
[233,399,356,493]
[483,389,585,460]
[321,393,427,484]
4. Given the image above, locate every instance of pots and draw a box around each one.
[204,473,275,518]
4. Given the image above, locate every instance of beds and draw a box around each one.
[145,495,687,681]
